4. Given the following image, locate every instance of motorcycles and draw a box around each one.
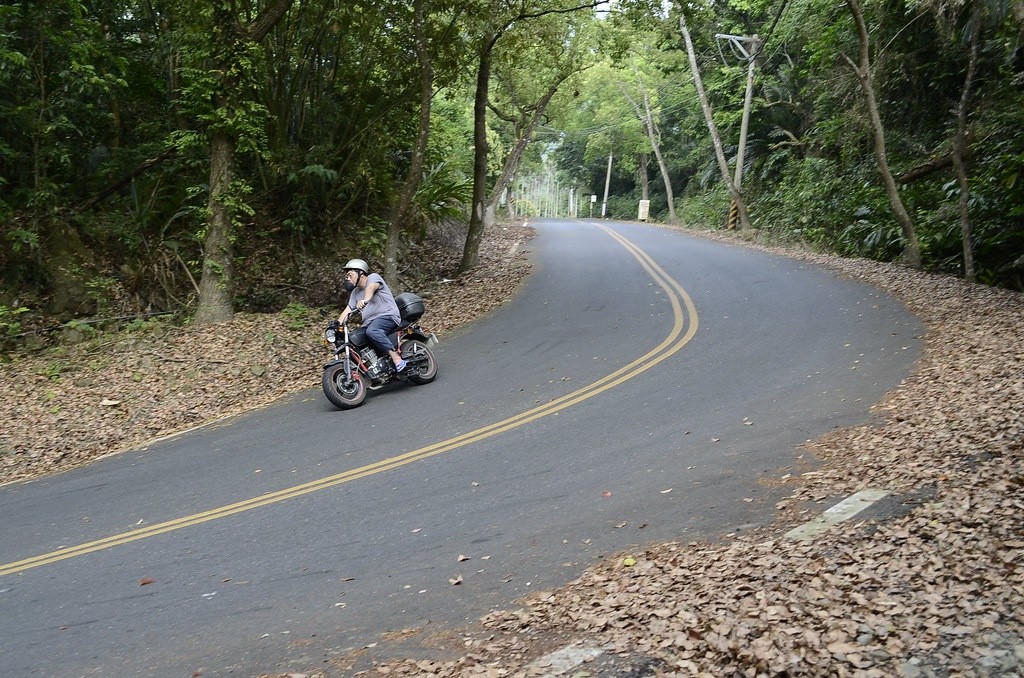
[321,292,439,411]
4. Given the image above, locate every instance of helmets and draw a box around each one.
[342,258,368,275]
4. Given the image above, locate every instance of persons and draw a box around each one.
[337,259,406,372]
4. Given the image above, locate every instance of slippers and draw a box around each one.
[396,361,406,372]
